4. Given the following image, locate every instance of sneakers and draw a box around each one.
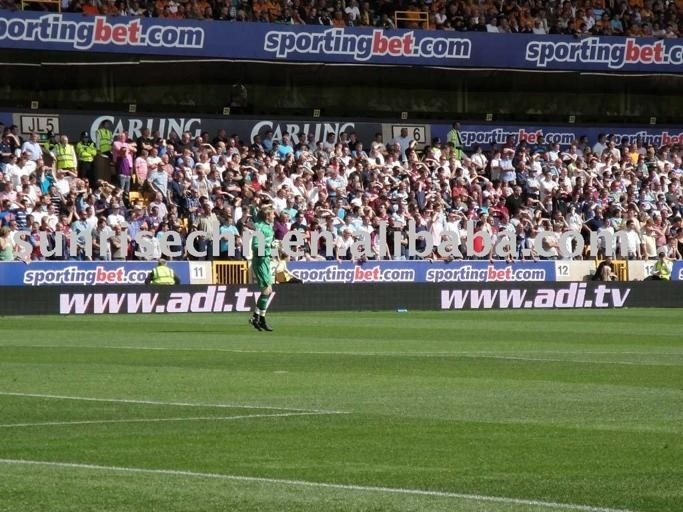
[249,315,273,331]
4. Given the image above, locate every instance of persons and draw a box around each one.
[246,205,276,332]
[0,0,683,283]
